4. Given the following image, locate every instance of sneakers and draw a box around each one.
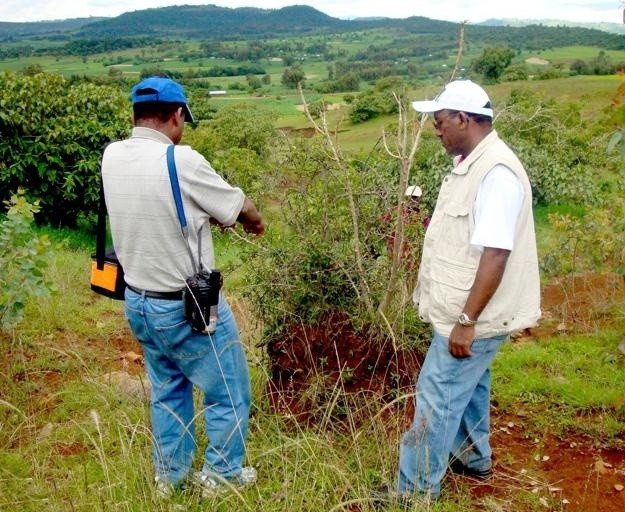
[158,466,258,499]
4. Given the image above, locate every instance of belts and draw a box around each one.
[126,285,183,300]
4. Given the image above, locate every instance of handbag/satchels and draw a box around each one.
[90,251,125,300]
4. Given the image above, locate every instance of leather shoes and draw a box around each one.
[447,453,493,481]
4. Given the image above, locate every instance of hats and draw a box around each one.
[413,81,494,117]
[132,78,194,123]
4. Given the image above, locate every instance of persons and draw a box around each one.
[368,80,541,500]
[101,75,266,502]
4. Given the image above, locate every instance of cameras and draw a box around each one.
[184,270,222,336]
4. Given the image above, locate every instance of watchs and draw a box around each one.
[457,311,477,327]
